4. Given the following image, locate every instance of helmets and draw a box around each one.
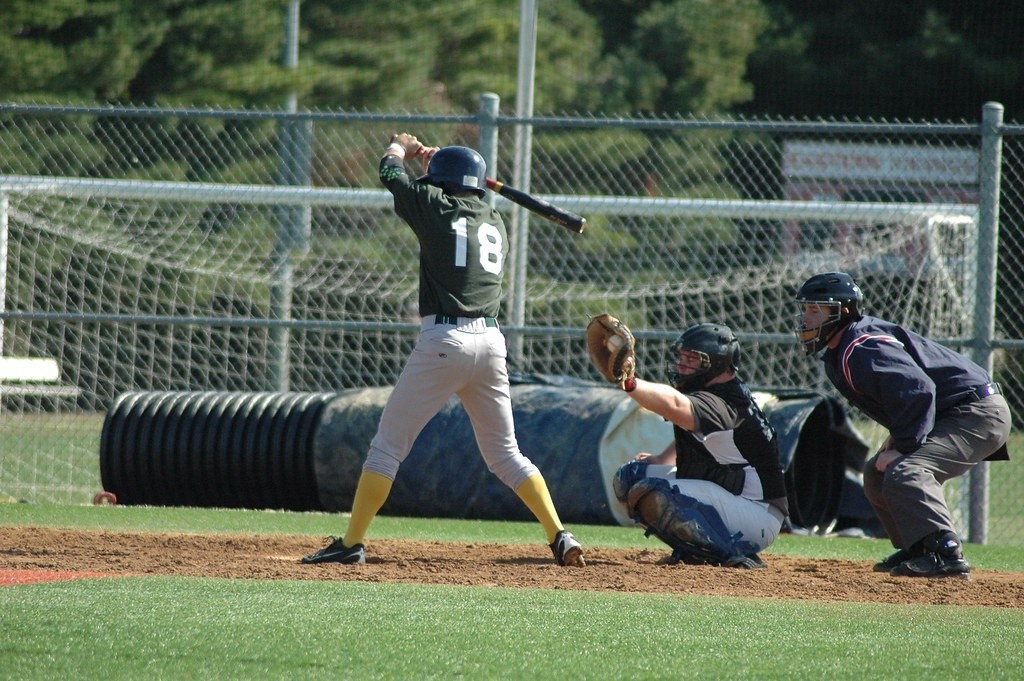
[416,146,486,198]
[793,272,863,356]
[666,322,740,392]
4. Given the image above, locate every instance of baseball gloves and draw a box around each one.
[586,315,636,385]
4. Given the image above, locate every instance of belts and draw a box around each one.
[936,382,1002,412]
[435,315,497,328]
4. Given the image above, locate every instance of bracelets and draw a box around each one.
[388,143,408,156]
[625,378,638,394]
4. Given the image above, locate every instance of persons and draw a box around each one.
[795,271,1012,577]
[301,132,587,565]
[588,314,790,567]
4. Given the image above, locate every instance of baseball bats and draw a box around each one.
[389,131,588,235]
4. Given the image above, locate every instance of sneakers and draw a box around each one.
[890,532,970,580]
[872,542,930,572]
[667,549,720,566]
[303,535,366,565]
[735,553,766,570]
[548,530,586,568]
